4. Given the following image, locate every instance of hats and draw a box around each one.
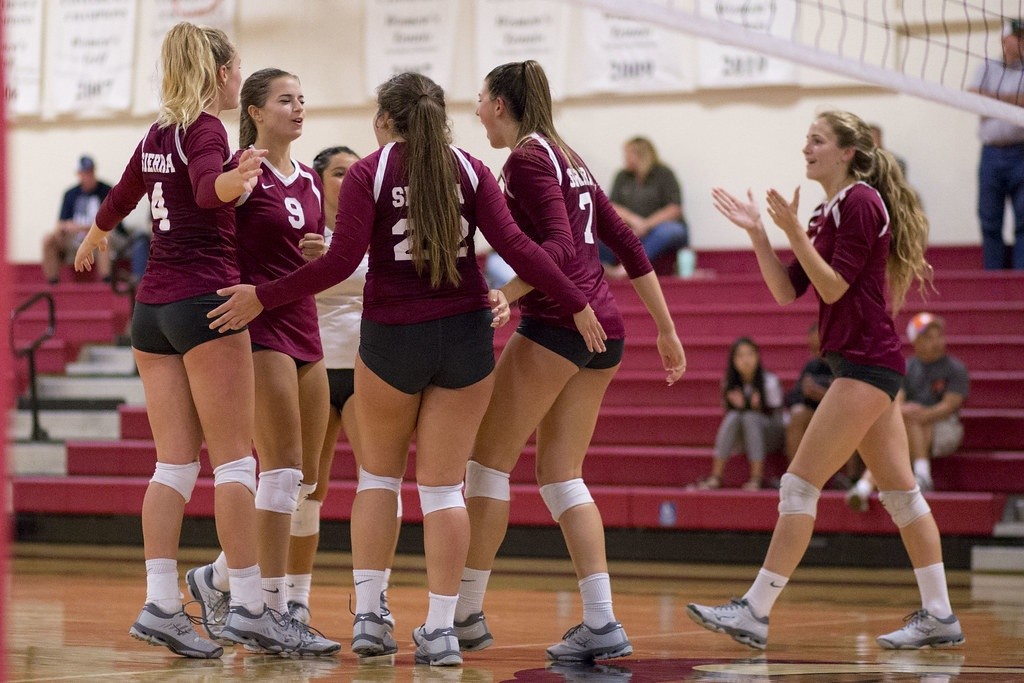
[905,313,945,343]
[74,157,92,170]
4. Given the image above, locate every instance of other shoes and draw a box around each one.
[848,488,869,512]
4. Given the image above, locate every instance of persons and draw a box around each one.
[846,312,967,509]
[284,145,403,627]
[207,74,607,669]
[687,112,965,651]
[692,336,785,490]
[599,138,687,278]
[74,22,303,659]
[413,61,686,662]
[42,157,130,282]
[184,69,341,658]
[775,326,857,490]
[966,15,1024,270]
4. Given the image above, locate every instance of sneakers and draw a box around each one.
[351,613,399,657]
[284,597,310,629]
[547,662,632,683]
[414,627,461,666]
[544,622,633,666]
[157,653,493,683]
[414,610,493,653]
[244,617,341,659]
[876,609,967,650]
[685,595,770,651]
[220,604,302,657]
[131,601,224,658]
[185,564,233,639]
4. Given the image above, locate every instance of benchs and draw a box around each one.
[0,247,1024,564]
